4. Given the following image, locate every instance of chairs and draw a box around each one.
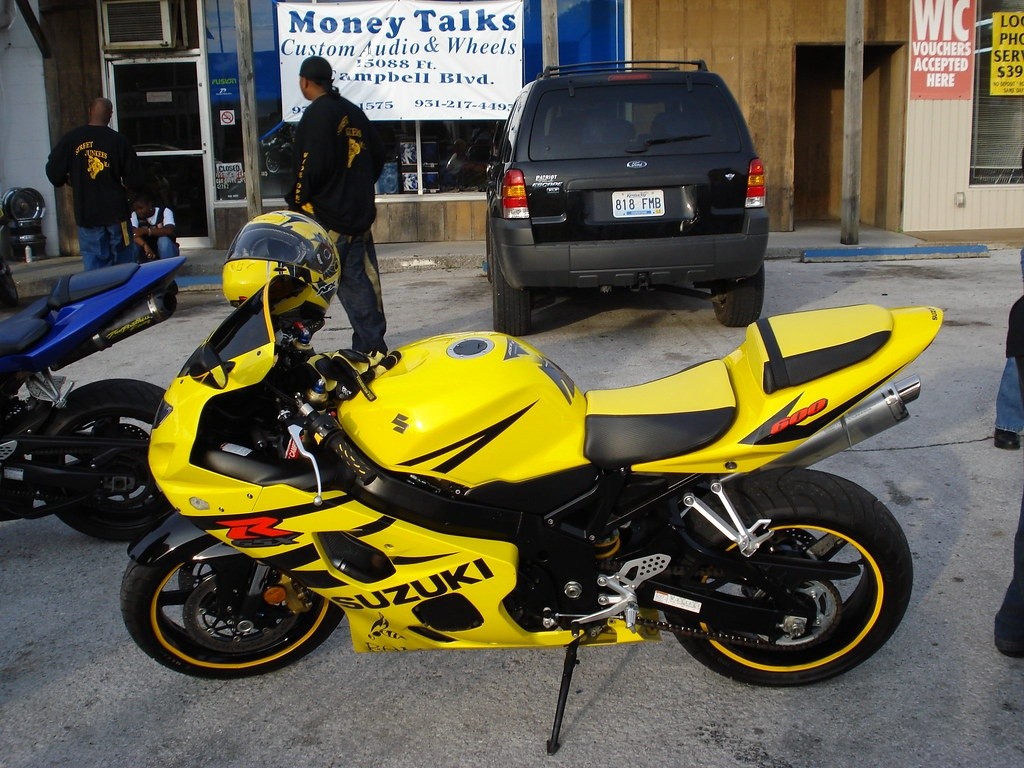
[650,113,696,135]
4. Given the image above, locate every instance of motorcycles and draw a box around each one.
[263,121,301,178]
[0,243,188,546]
[119,266,945,757]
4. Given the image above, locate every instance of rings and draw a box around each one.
[134,234,135,236]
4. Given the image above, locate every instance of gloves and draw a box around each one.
[302,345,401,402]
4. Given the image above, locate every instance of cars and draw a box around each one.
[123,144,242,212]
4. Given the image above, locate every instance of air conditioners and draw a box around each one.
[101,0,172,50]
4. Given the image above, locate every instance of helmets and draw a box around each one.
[222,210,342,316]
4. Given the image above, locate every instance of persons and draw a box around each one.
[128,191,179,263]
[287,56,389,357]
[993,249,1024,661]
[46,97,137,271]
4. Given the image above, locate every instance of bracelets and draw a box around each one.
[142,242,146,246]
[148,227,151,236]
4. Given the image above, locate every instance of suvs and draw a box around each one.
[446,120,491,191]
[487,59,770,334]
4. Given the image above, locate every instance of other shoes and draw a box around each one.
[994,635,1024,656]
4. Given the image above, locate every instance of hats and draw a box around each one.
[299,56,335,86]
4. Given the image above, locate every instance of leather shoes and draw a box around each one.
[994,427,1020,450]
